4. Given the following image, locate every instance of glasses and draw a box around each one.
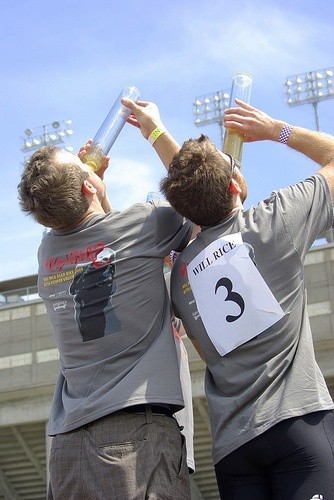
[224,153,238,192]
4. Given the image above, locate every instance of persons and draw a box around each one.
[17,96,201,500]
[155,98,334,500]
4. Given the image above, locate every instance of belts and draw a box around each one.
[120,401,176,418]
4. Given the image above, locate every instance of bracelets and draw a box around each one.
[148,125,167,147]
[279,122,294,146]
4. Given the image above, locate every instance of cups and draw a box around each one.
[82,83,142,171]
[223,72,253,169]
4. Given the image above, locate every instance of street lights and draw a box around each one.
[190,87,231,146]
[19,120,74,239]
[282,67,332,132]
